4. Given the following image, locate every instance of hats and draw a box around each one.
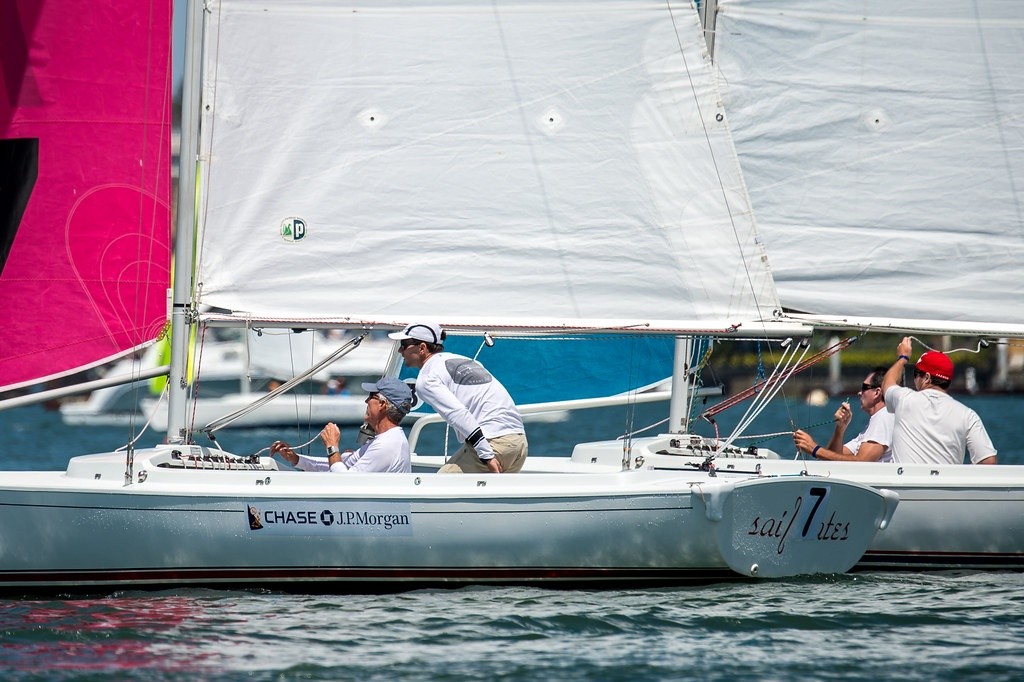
[904,351,954,381]
[361,375,412,414]
[388,319,444,345]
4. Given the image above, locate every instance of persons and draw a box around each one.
[387,323,529,473]
[793,366,895,462]
[880,336,998,464]
[322,377,351,395]
[270,376,413,473]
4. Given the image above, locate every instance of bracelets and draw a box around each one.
[812,446,820,458]
[897,355,909,362]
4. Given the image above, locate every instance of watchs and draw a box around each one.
[326,446,339,456]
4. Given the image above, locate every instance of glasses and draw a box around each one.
[401,341,419,347]
[914,369,923,377]
[862,382,876,392]
[370,392,380,399]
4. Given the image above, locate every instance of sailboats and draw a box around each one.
[298,1,1023,572]
[1,0,814,590]
[56,2,724,433]
